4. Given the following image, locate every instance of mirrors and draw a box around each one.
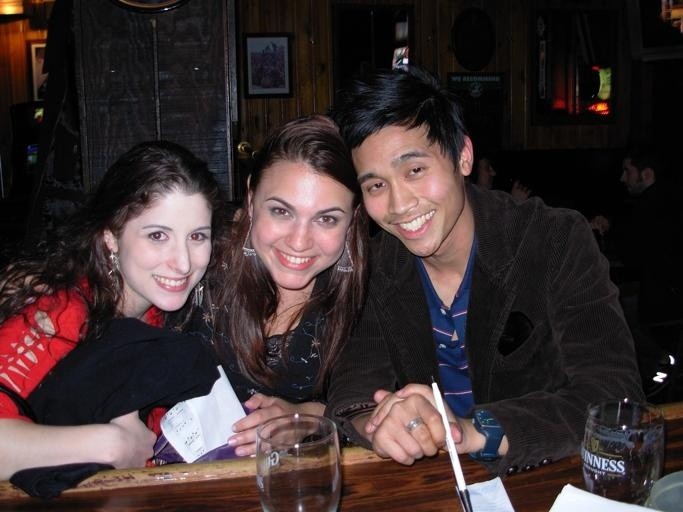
[526,9,616,126]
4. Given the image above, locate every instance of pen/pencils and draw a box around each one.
[429,375,473,512]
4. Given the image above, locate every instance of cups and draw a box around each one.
[256,415,342,512]
[581,401,665,505]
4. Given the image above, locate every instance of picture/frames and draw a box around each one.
[109,0,190,12]
[627,0,681,60]
[25,38,47,102]
[447,70,505,133]
[240,32,295,100]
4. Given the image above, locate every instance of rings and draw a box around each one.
[406,417,424,433]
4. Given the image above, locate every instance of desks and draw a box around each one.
[0,403,682,511]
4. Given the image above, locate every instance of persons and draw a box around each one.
[324,70,646,478]
[604,148,683,397]
[1,141,219,481]
[197,112,367,458]
[478,158,532,201]
